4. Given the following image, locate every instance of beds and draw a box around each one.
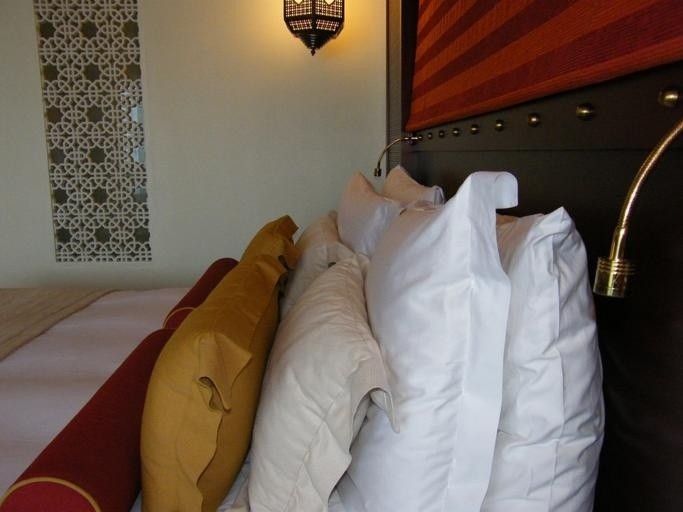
[1,60,682,512]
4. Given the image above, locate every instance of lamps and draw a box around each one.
[374,132,415,176]
[593,118,682,299]
[284,0,345,56]
